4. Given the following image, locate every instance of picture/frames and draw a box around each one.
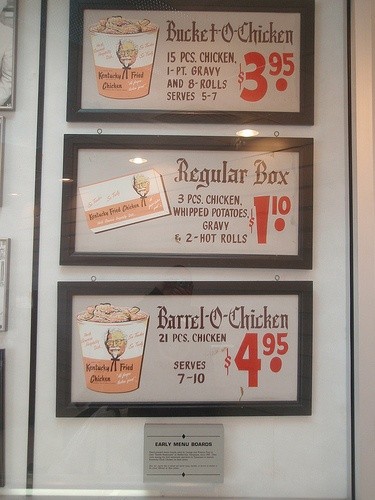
[60,132,316,268]
[54,279,313,415]
[1,1,23,487]
[65,1,314,124]
